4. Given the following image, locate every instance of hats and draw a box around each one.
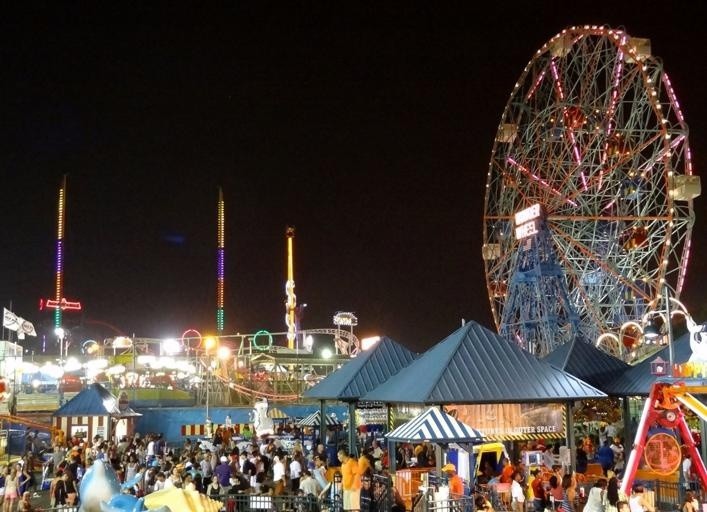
[441,464,456,472]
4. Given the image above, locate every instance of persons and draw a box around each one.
[1,414,707,512]
